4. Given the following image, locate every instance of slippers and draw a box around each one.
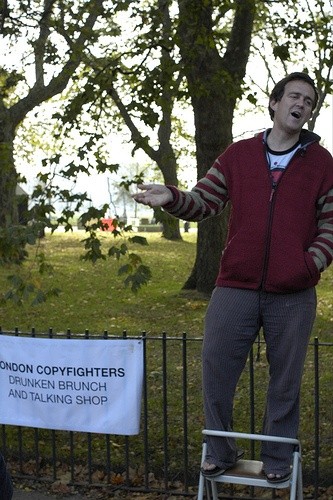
[266,475,291,484]
[202,448,245,478]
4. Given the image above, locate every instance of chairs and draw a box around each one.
[198,429,303,500]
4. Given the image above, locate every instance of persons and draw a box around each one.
[132,72,333,484]
